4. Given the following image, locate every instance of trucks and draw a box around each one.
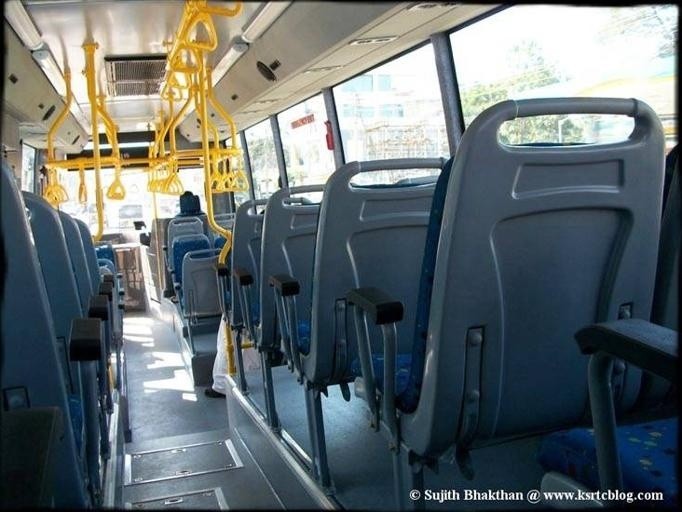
[88,203,108,228]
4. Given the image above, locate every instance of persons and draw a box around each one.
[171,191,209,304]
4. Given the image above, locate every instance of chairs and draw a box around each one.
[166,96,680,510]
[0,155,126,508]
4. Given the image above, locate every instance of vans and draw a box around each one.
[119,205,143,230]
